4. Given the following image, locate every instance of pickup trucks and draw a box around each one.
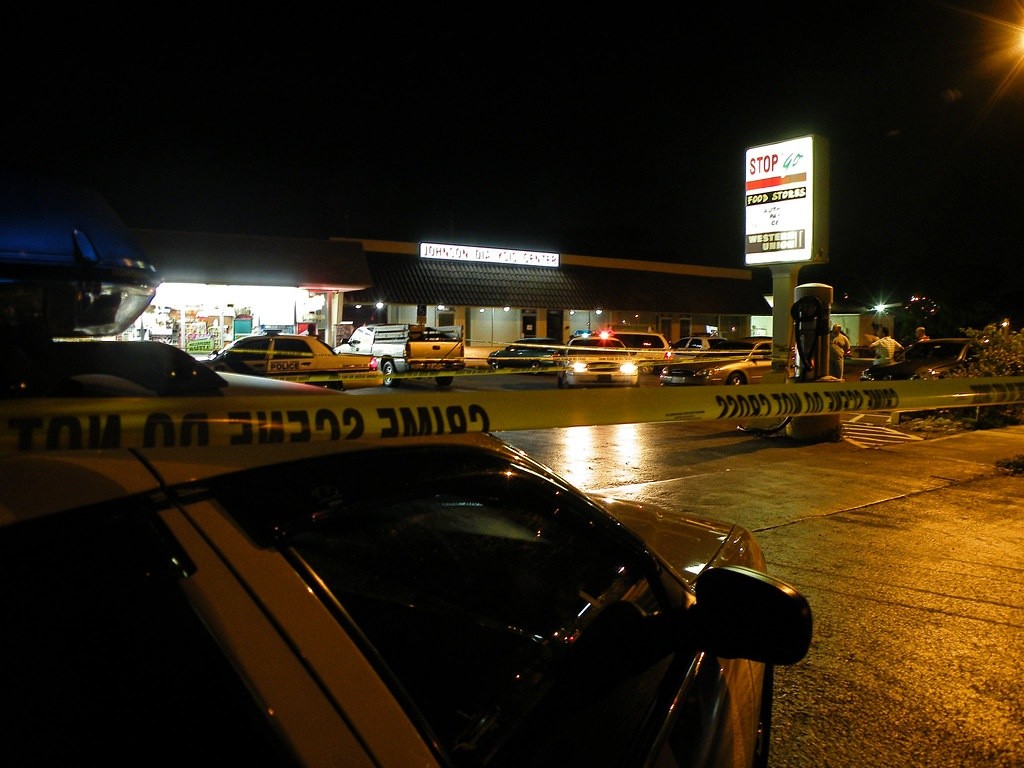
[331,322,465,388]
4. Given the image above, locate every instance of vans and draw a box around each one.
[609,331,674,373]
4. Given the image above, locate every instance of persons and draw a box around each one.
[829,323,931,381]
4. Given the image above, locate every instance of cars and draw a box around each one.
[487,337,565,375]
[860,337,985,382]
[2,398,812,768]
[193,329,384,394]
[671,337,728,352]
[0,434,814,768]
[558,335,640,388]
[11,336,347,427]
[658,338,797,390]
[569,330,592,339]
